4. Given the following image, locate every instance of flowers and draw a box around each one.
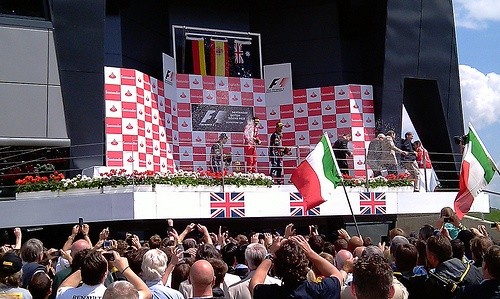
[13,170,274,193]
[338,173,412,186]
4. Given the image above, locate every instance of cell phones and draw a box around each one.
[79,218,83,228]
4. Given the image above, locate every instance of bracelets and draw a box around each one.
[121,266,130,274]
[83,233,89,236]
[67,236,74,240]
[265,255,274,262]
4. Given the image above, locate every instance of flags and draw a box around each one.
[290,131,344,209]
[289,192,321,217]
[209,38,230,77]
[233,39,252,78]
[183,36,207,76]
[359,192,387,214]
[210,192,246,218]
[454,122,500,221]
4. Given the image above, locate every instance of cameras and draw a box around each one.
[444,218,453,222]
[104,241,111,248]
[102,252,115,261]
[491,223,497,229]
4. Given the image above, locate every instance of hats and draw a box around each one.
[219,133,228,139]
[0,253,23,278]
[276,122,285,127]
[440,207,454,218]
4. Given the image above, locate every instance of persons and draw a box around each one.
[0,207,500,299]
[208,117,432,192]
